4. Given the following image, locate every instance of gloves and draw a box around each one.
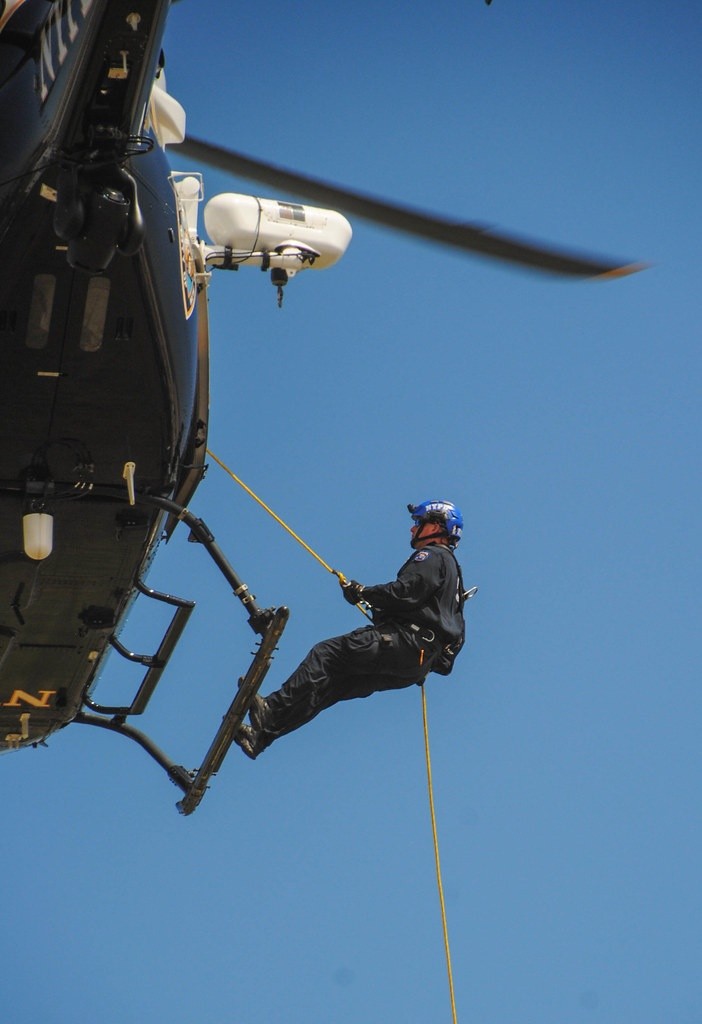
[342,581,362,606]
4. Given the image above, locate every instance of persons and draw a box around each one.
[222,499,466,760]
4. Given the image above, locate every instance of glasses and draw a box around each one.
[415,519,422,526]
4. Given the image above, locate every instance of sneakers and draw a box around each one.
[223,715,264,760]
[238,676,266,731]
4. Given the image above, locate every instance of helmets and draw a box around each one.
[411,499,464,551]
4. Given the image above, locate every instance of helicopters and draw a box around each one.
[0,0,657,806]
